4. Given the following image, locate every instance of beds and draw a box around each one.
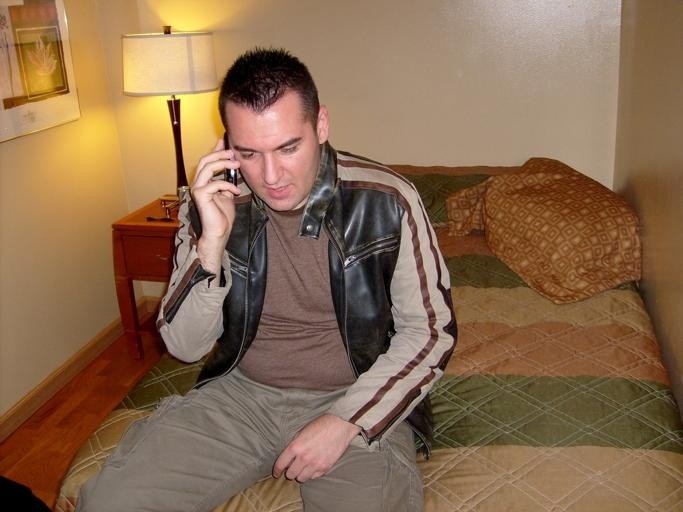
[54,166,683,511]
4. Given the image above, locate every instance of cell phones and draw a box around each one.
[221,131,239,187]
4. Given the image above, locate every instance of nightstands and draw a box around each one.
[113,194,178,361]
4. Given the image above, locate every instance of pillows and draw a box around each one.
[447,157,641,304]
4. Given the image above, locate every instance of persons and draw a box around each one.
[77,45,457,512]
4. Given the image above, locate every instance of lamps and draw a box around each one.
[121,26,220,218]
